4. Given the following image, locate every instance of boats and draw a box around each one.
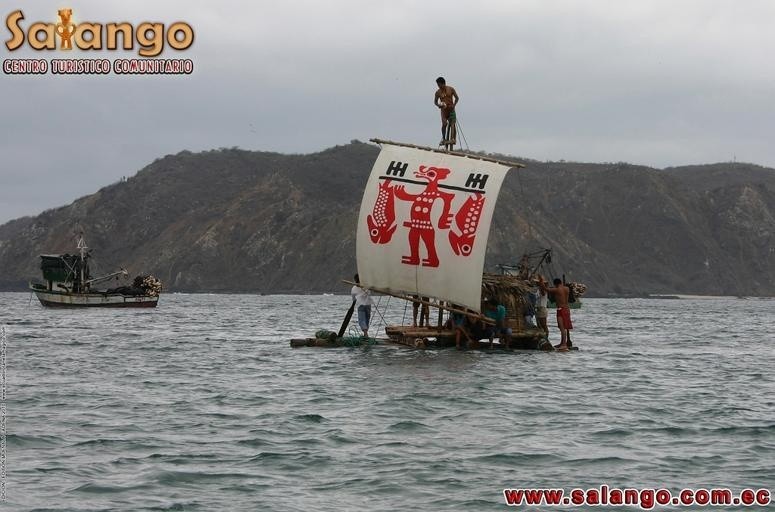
[28,230,159,307]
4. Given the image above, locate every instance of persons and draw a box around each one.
[351,273,372,337]
[434,76,460,146]
[120,264,129,281]
[443,296,513,351]
[517,265,527,279]
[537,274,573,349]
[411,295,430,327]
[535,276,549,338]
